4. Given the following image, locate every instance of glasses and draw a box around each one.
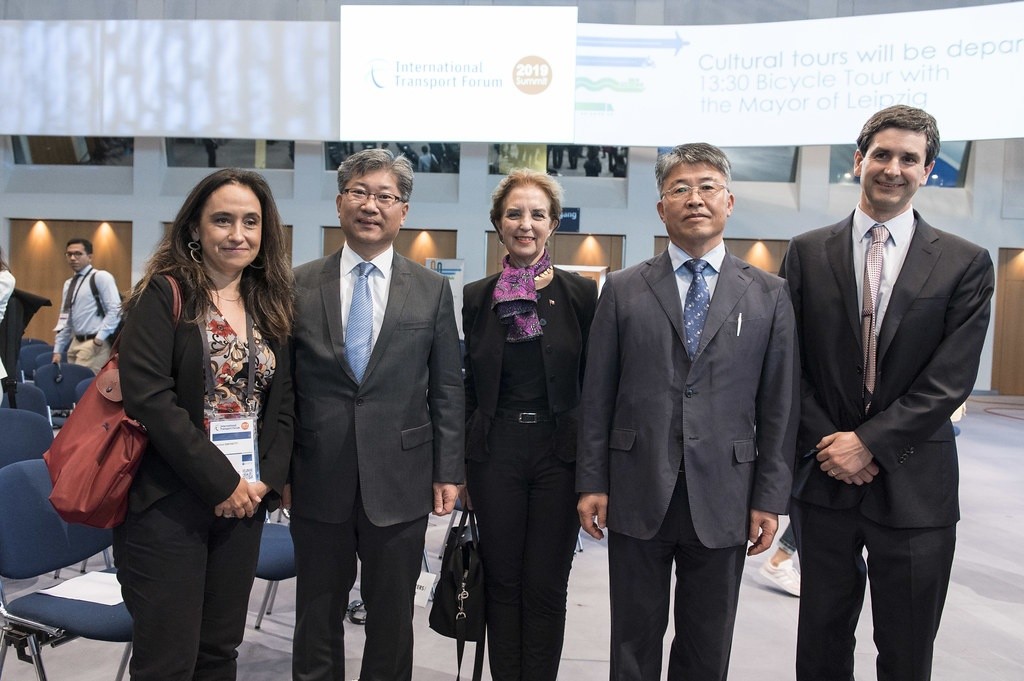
[65,251,83,259]
[660,183,731,199]
[342,188,406,208]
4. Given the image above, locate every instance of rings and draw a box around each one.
[831,469,837,475]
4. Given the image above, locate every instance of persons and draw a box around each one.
[52,238,123,377]
[0,246,17,407]
[489,142,627,178]
[754,104,993,681]
[578,143,799,681]
[203,138,219,167]
[458,168,598,681]
[325,141,460,173]
[111,148,294,681]
[277,148,468,681]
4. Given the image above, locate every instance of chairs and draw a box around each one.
[423,497,465,601]
[0,338,297,681]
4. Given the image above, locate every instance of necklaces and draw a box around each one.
[210,290,241,301]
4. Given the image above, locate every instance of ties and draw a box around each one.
[863,225,889,395]
[56,273,81,333]
[344,263,376,385]
[683,258,712,359]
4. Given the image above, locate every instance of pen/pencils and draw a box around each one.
[737,313,742,337]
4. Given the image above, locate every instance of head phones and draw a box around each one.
[53,361,63,383]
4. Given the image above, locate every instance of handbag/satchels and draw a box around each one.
[430,504,488,681]
[43,351,152,531]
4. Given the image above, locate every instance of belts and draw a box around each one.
[74,334,97,342]
[498,409,549,425]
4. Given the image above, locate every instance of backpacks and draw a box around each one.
[90,271,126,348]
[430,154,442,173]
